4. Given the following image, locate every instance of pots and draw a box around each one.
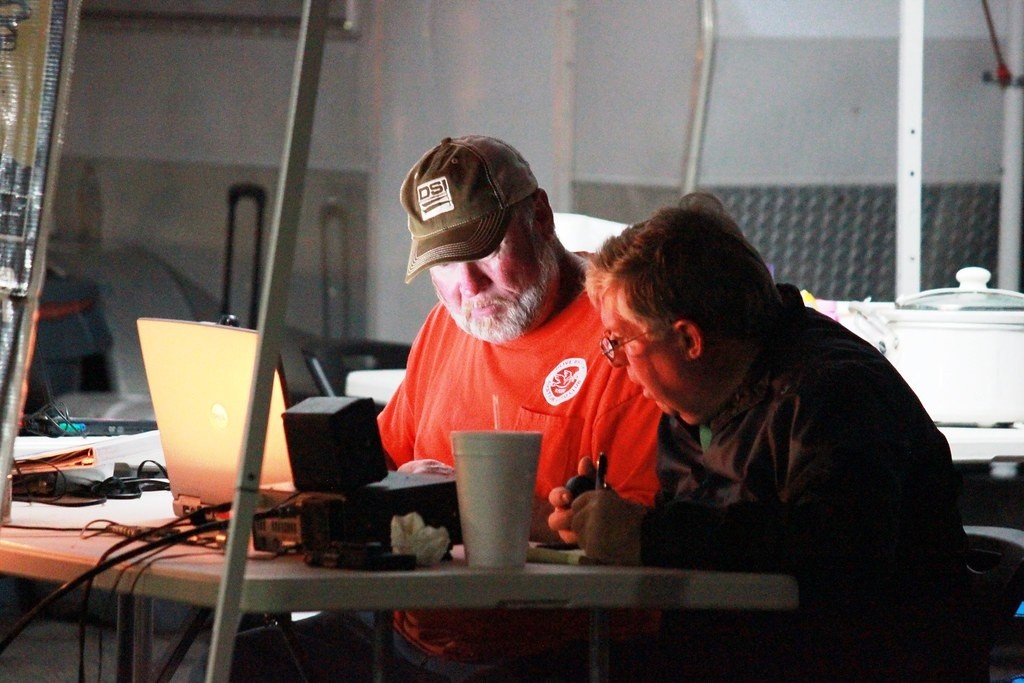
[850,267,1024,424]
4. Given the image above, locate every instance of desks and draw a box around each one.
[0,488,803,683]
[937,426,1024,462]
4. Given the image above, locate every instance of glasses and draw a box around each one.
[598,330,651,360]
[113,460,169,479]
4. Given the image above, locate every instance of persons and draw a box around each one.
[458,191,991,683]
[184,134,666,683]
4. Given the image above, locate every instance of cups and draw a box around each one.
[448,429,544,571]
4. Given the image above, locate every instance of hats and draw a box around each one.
[400,135,539,285]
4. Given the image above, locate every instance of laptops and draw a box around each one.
[136,317,294,527]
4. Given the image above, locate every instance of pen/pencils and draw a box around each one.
[595,451,607,491]
[311,357,335,397]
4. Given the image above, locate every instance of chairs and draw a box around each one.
[304,351,337,396]
